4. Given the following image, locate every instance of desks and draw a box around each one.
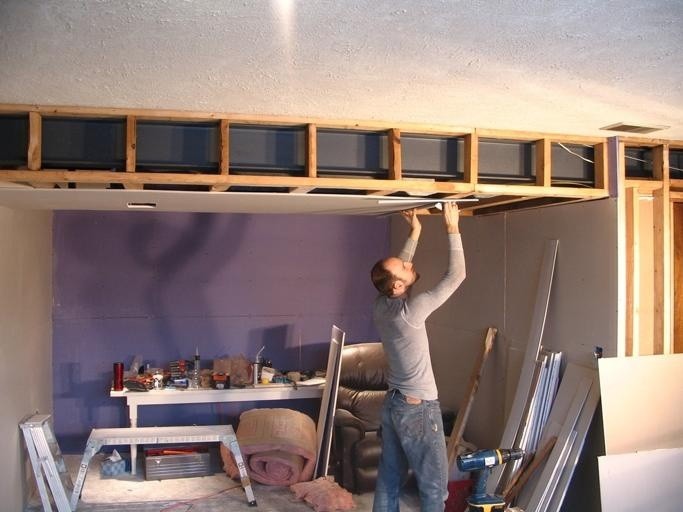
[109,379,324,474]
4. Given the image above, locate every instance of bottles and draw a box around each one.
[252,346,265,384]
[193,344,201,385]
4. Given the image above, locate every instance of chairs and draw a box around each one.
[334,343,415,493]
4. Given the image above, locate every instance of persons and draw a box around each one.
[372,202,467,511]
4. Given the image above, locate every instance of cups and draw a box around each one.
[112,360,122,391]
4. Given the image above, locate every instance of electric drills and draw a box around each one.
[456,448,536,511]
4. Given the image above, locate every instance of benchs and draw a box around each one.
[74,425,258,510]
